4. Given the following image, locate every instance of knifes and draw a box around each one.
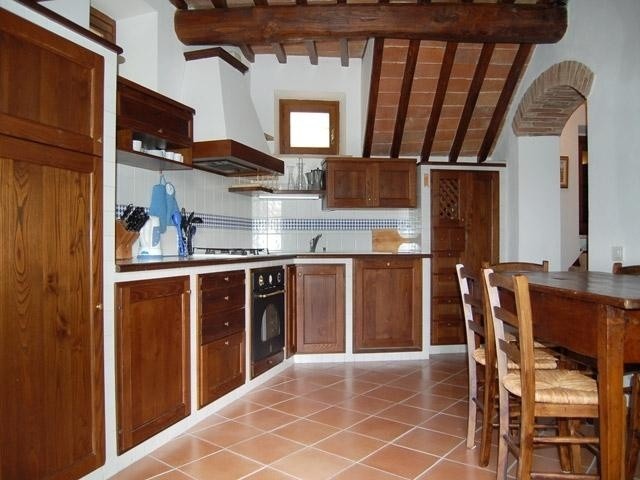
[117,206,153,231]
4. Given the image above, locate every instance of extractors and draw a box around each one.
[195,140,285,185]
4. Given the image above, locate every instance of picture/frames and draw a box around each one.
[559,155,569,189]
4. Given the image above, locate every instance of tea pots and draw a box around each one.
[304,167,326,195]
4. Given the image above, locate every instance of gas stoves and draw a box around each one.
[198,242,269,260]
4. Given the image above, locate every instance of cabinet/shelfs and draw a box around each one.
[0,8,105,478]
[318,155,417,211]
[348,258,423,355]
[430,170,499,344]
[193,267,249,413]
[286,261,345,358]
[112,271,197,460]
[116,75,198,175]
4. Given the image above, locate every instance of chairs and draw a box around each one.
[454,260,640,480]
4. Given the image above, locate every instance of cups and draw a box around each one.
[132,138,186,164]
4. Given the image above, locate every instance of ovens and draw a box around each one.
[242,264,291,371]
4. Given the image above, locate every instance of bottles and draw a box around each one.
[287,157,307,195]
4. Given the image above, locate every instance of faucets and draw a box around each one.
[311,233,322,253]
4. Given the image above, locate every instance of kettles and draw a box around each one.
[137,214,162,262]
